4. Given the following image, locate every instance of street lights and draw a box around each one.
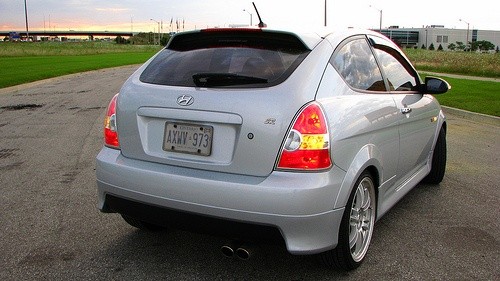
[459,18,469,52]
[369,4,382,33]
[243,9,252,27]
[150,18,161,45]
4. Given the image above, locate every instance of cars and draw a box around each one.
[94,24,451,273]
